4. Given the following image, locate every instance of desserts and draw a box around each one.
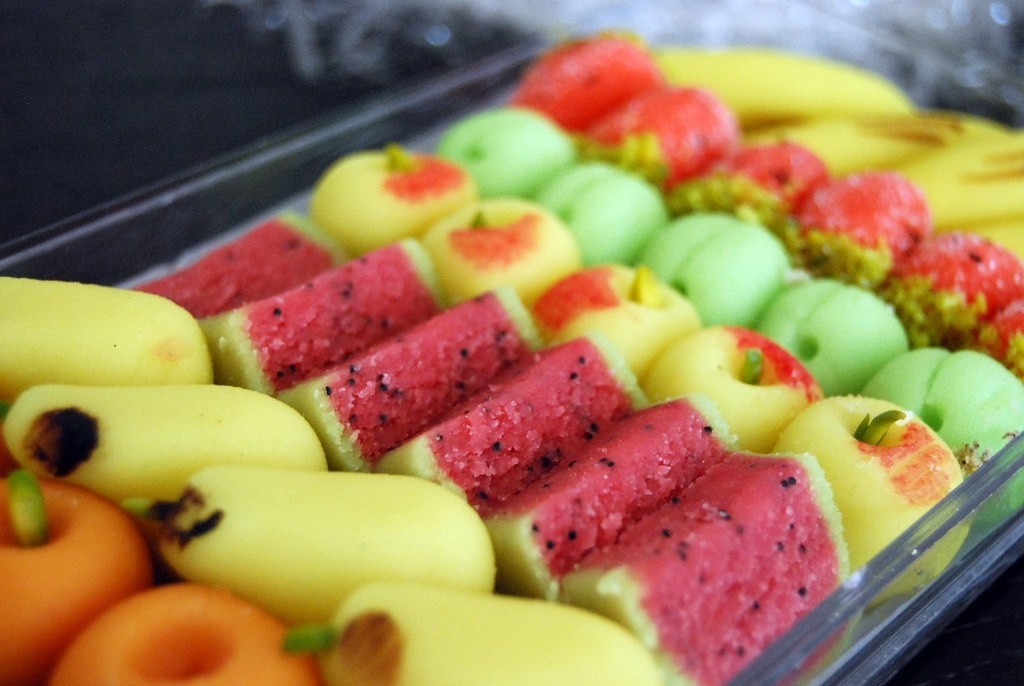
[0,33,1024,686]
